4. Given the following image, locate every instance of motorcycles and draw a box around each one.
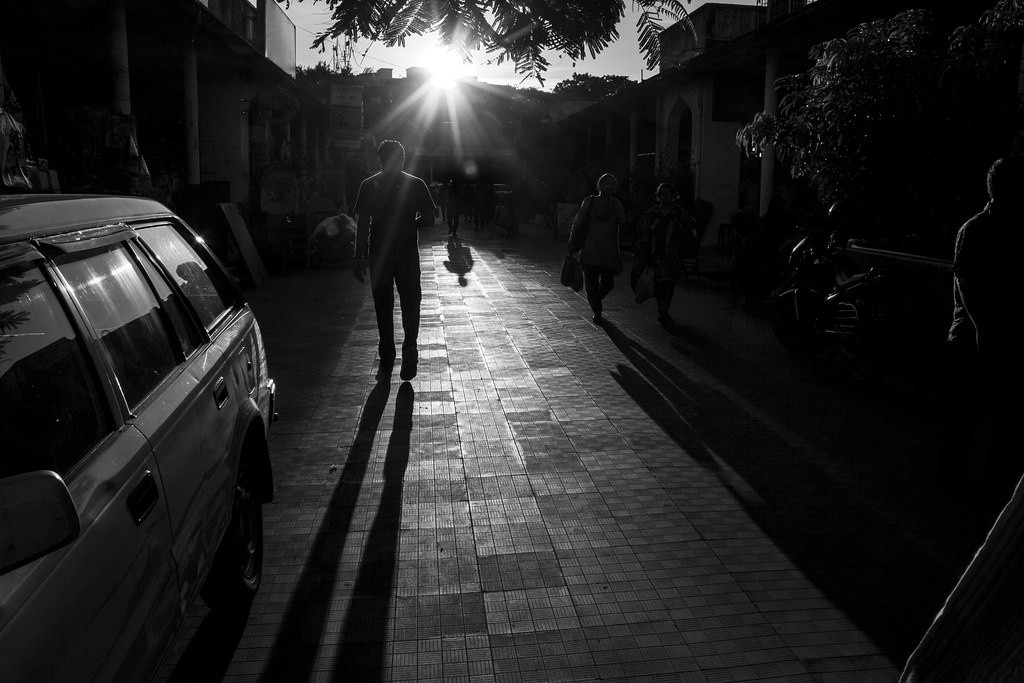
[771,199,885,366]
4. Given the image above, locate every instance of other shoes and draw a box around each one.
[400,344,417,380]
[658,313,672,322]
[448,229,451,234]
[480,225,486,231]
[378,352,397,374]
[473,226,479,230]
[592,313,602,323]
[453,232,457,237]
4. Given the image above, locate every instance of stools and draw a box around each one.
[716,223,735,258]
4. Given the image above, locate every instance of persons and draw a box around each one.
[439,163,522,238]
[353,140,436,381]
[936,157,1024,522]
[568,174,690,322]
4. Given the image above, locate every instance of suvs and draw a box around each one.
[0,187,274,683]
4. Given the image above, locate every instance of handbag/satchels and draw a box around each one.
[630,263,667,301]
[496,208,512,229]
[560,255,585,292]
[568,194,595,249]
[677,205,700,260]
